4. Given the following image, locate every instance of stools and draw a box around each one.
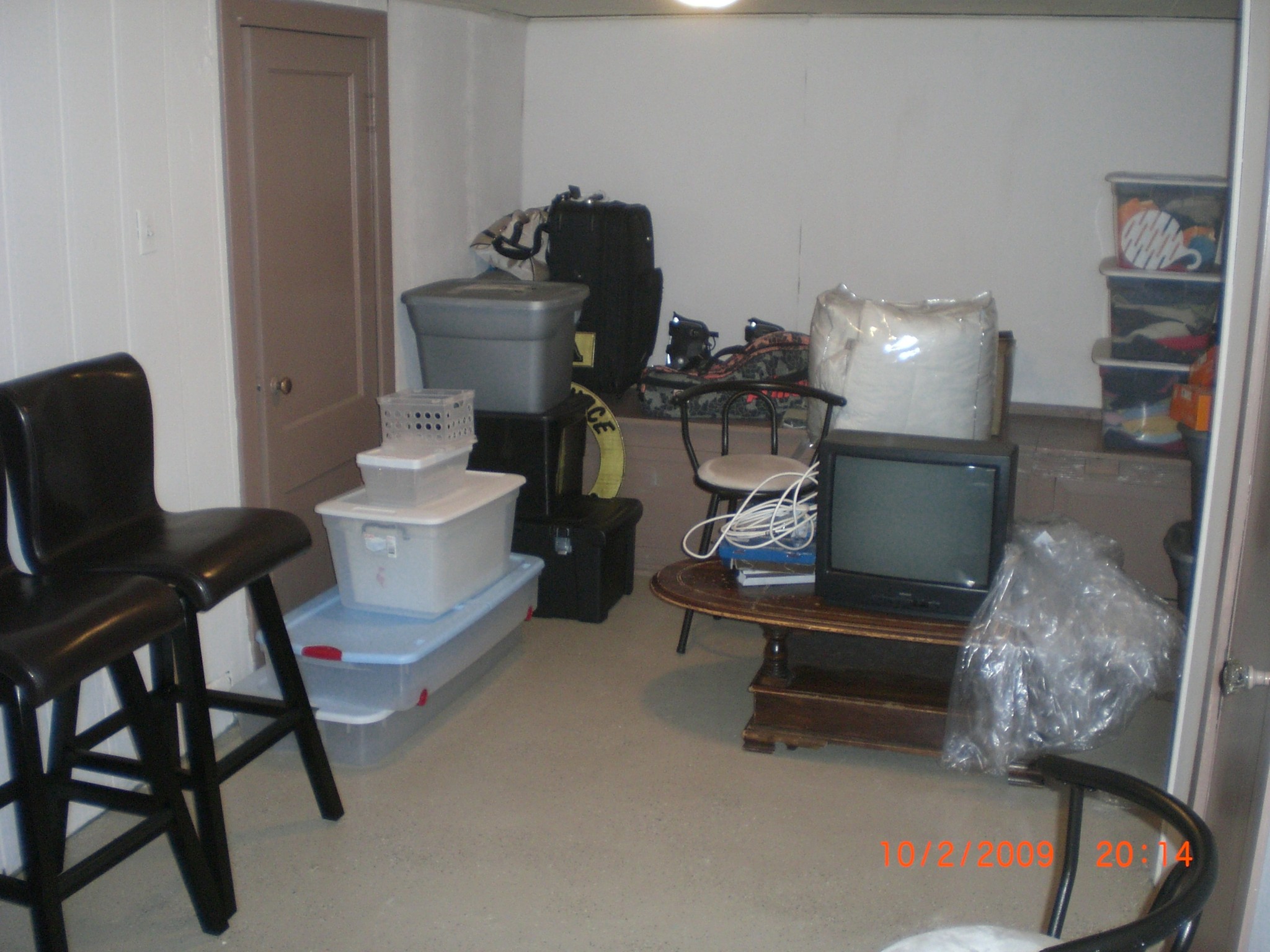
[0,351,344,952]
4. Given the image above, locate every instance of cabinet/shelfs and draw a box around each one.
[648,556,1045,788]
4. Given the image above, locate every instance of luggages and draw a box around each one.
[545,186,663,399]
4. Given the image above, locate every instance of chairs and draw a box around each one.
[882,755,1219,952]
[671,380,847,655]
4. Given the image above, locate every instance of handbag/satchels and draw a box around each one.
[637,330,810,422]
[469,205,551,282]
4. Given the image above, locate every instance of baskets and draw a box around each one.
[376,388,474,446]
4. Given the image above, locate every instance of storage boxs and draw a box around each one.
[1091,170,1229,455]
[230,278,644,769]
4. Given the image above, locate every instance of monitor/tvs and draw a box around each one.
[815,429,1019,624]
